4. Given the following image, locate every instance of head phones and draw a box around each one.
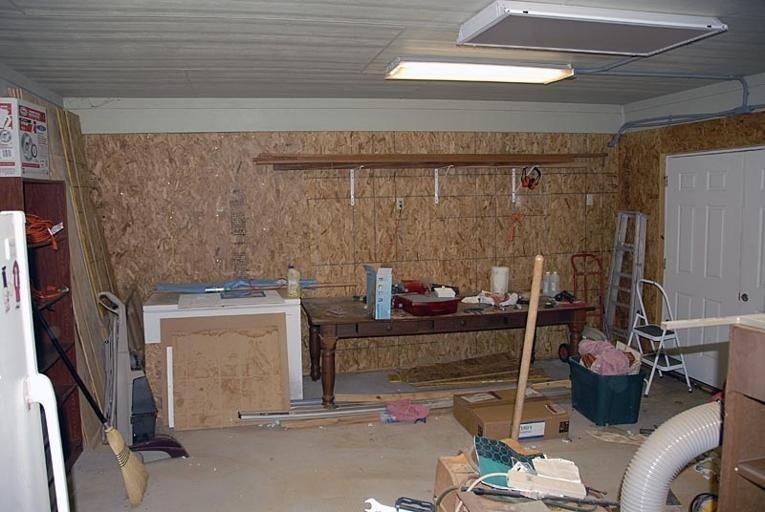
[521,167,541,190]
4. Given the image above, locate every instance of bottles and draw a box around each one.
[543,271,559,298]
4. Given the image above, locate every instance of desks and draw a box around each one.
[302,291,595,410]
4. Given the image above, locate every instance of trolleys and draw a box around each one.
[558,251,614,362]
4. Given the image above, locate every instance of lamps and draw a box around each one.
[458,0,729,58]
[384,56,576,85]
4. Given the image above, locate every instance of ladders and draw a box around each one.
[602,210,647,351]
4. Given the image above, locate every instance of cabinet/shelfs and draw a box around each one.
[0,177,83,486]
[715,320,765,512]
[625,279,693,398]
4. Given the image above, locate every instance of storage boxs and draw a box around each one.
[0,97,50,177]
[453,388,570,447]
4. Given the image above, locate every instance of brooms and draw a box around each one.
[34,309,149,505]
